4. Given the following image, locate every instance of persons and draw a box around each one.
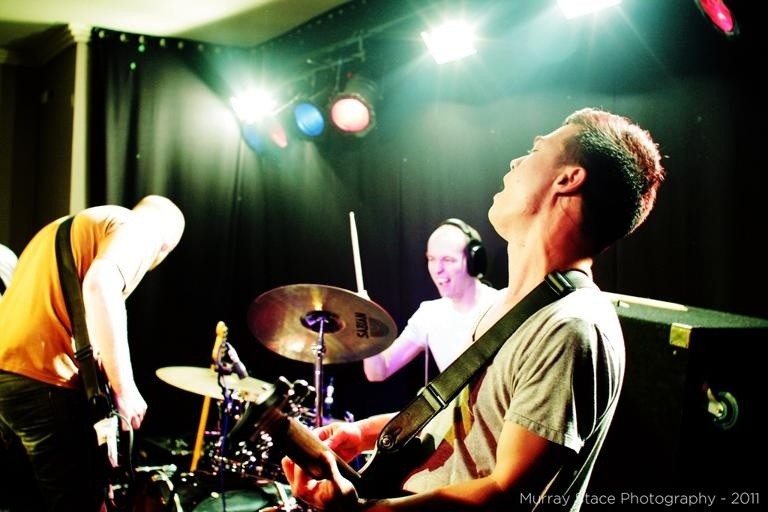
[0,192,187,511]
[353,217,507,384]
[279,107,667,511]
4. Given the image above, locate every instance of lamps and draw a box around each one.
[236,35,390,157]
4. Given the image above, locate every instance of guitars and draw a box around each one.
[83,361,123,468]
[222,376,437,512]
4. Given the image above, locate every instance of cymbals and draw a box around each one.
[157,366,279,403]
[248,282,397,365]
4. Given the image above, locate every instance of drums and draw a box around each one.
[168,472,282,512]
